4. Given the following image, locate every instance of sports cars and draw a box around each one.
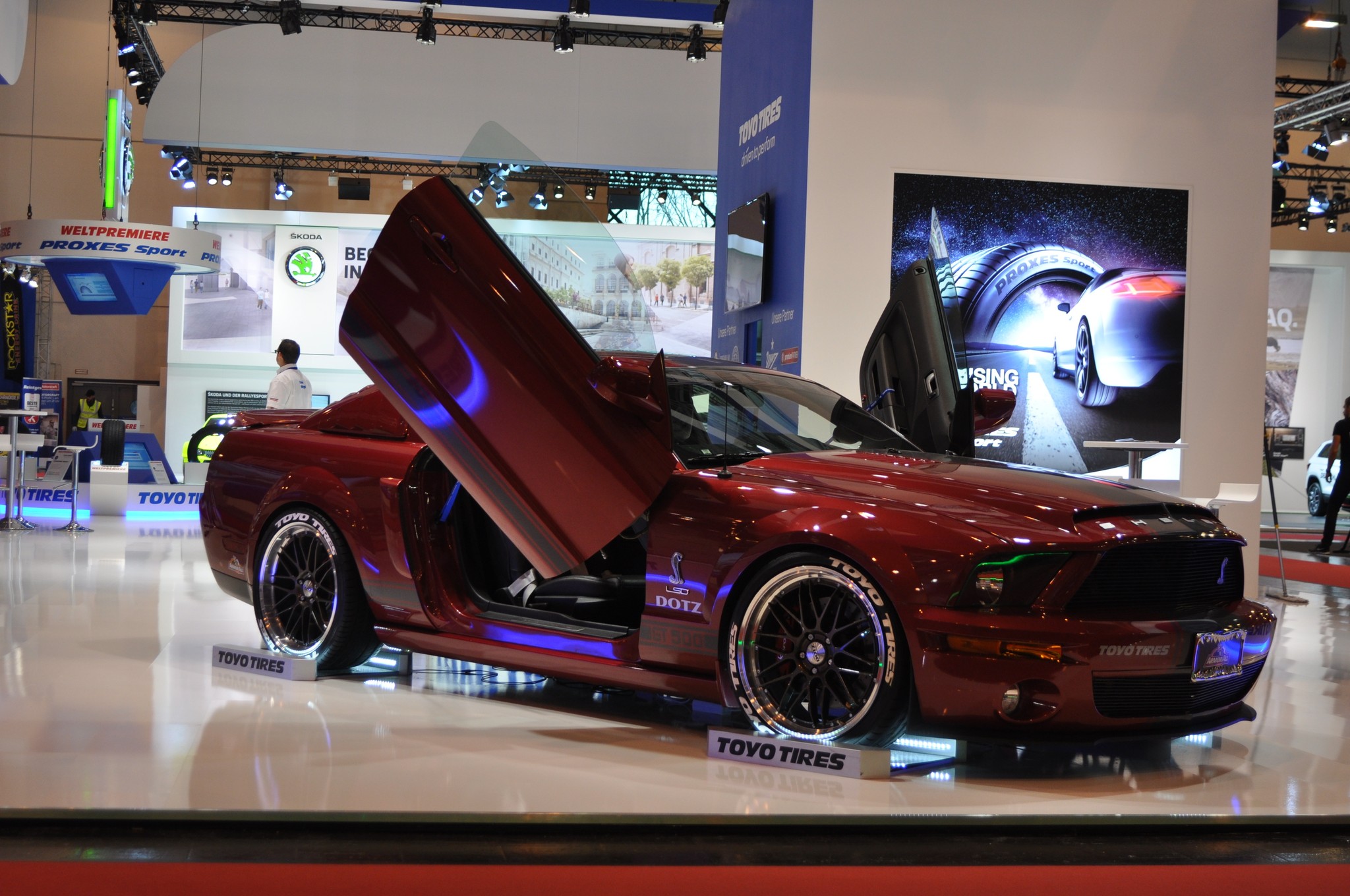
[1050,267,1185,408]
[196,177,1277,750]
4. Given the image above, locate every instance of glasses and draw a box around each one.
[275,349,280,354]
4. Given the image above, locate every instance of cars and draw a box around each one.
[1305,440,1350,517]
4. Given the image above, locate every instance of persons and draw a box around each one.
[265,339,312,409]
[1311,397,1350,555]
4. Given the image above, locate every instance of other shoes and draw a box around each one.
[1308,544,1330,554]
[1333,548,1350,557]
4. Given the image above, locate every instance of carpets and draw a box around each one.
[1259,554,1350,589]
[1260,532,1350,542]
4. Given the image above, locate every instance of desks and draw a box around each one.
[0,410,48,531]
[1084,440,1189,480]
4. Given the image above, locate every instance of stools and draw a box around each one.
[1181,483,1258,519]
[0,434,99,532]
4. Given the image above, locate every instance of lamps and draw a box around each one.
[206,164,234,186]
[278,0,302,36]
[657,187,668,204]
[1296,190,1345,233]
[5,262,39,288]
[553,0,589,54]
[273,169,295,201]
[1299,13,1347,28]
[415,0,442,45]
[691,190,701,205]
[112,0,158,107]
[468,161,597,210]
[1271,118,1341,174]
[170,155,195,188]
[686,24,706,62]
